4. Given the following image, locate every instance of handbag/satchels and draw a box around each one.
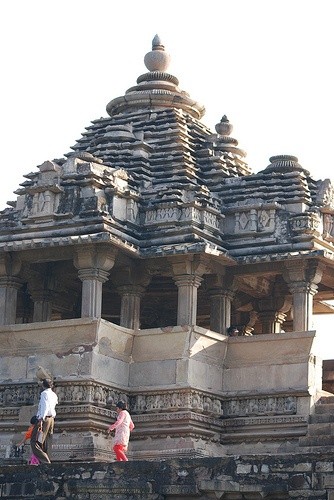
[36,420,44,445]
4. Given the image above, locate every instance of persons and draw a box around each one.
[227,327,238,336]
[30,378,58,463]
[108,401,135,462]
[15,415,41,465]
